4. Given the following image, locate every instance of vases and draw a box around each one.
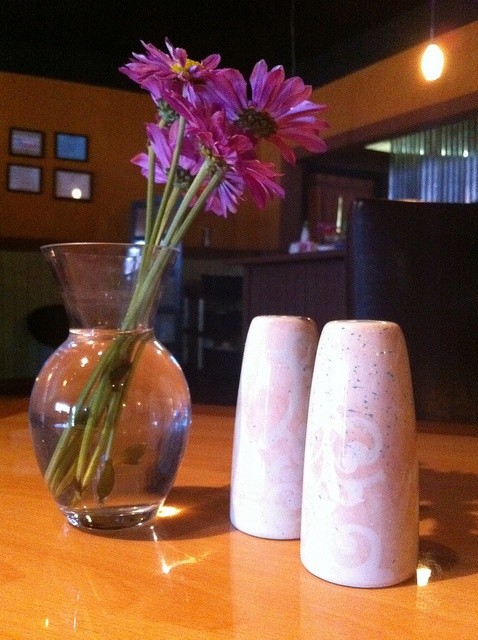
[27,239,193,535]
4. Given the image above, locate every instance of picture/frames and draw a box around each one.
[8,127,46,160]
[52,167,94,204]
[7,162,41,195]
[53,131,89,163]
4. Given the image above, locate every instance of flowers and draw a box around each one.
[42,34,334,490]
